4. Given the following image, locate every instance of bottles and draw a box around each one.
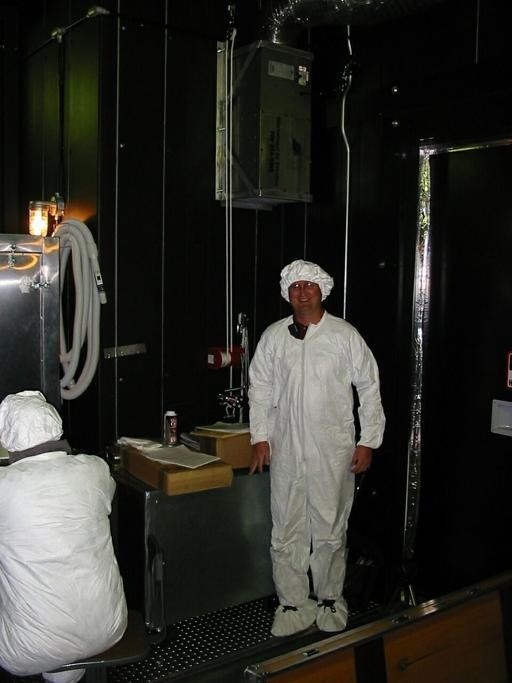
[27,200,51,238]
[163,410,178,448]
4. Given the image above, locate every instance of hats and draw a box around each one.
[278,258,335,303]
[0,388,67,453]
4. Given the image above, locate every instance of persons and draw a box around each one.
[246,259,387,638]
[0,391,129,681]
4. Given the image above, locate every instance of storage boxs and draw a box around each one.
[119,431,253,497]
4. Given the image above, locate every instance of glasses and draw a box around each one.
[291,281,319,289]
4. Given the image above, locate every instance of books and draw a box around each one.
[179,421,251,451]
